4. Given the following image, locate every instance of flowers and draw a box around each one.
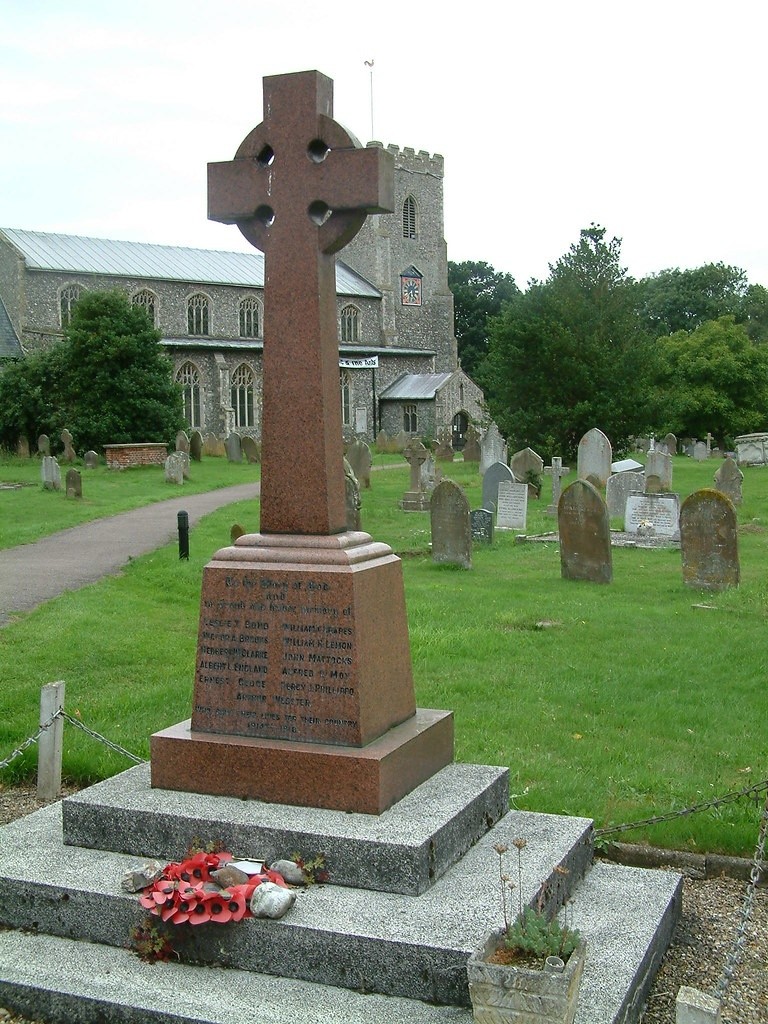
[493,837,584,957]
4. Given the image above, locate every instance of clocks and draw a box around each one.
[401,276,421,307]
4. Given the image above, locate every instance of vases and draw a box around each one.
[466,931,587,1024]
